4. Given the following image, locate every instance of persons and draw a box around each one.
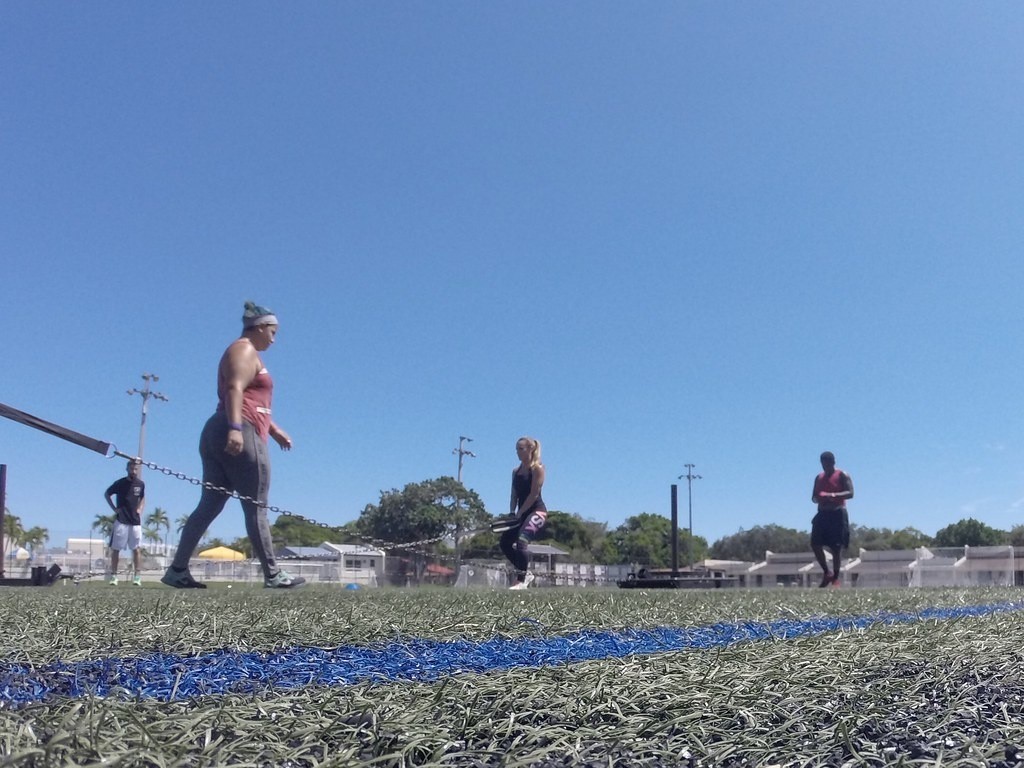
[104,459,146,586]
[497,436,548,591]
[161,301,305,589]
[810,451,855,589]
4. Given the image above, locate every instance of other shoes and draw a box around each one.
[832,579,840,588]
[819,572,834,587]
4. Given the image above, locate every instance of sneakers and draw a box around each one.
[109,575,117,585]
[524,574,534,585]
[509,582,527,590]
[133,575,141,586]
[264,572,305,588]
[161,567,207,589]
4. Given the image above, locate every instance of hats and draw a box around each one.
[243,301,278,327]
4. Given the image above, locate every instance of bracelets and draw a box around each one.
[226,422,242,432]
[832,492,835,499]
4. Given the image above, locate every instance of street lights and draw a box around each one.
[452,436,477,581]
[678,464,702,571]
[126,372,170,480]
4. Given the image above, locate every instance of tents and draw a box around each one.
[6,547,36,561]
[278,546,332,559]
[199,546,247,577]
